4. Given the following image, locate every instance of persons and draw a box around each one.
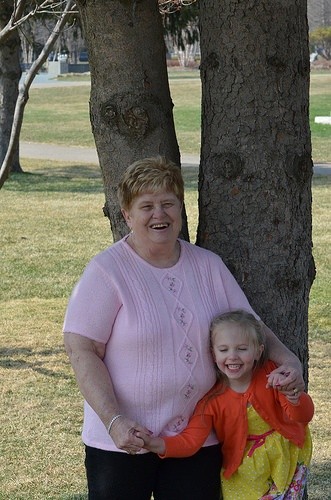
[133,311,314,500]
[63,156,306,500]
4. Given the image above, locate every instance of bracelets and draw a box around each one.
[108,415,122,434]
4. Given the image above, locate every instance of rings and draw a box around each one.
[293,388,298,395]
